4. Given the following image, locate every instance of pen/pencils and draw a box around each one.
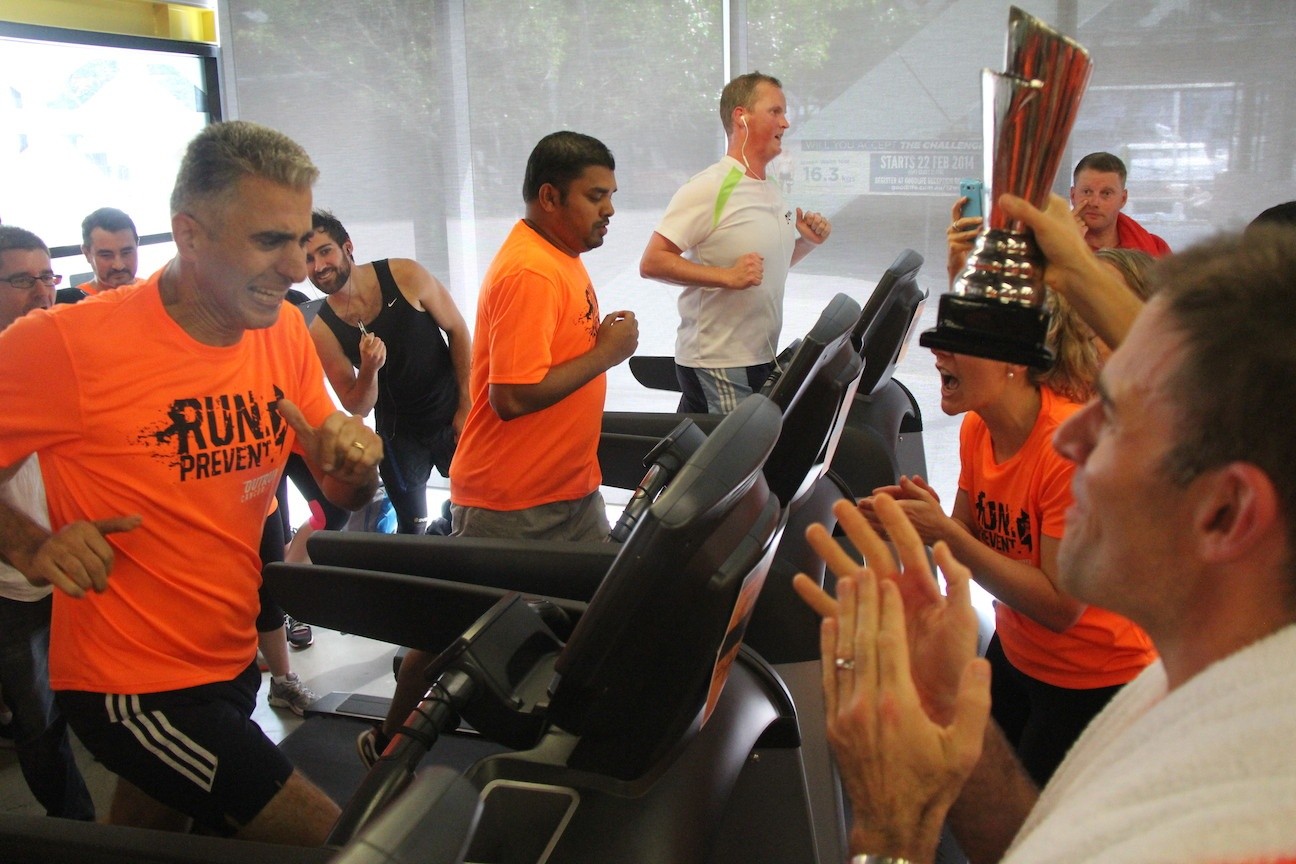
[356,319,368,338]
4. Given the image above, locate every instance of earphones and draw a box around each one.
[741,115,747,127]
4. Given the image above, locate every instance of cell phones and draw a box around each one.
[960,180,983,235]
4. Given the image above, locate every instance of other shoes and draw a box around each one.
[1,716,17,749]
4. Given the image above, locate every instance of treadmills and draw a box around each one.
[1,390,819,864]
[600,249,930,555]
[262,294,868,801]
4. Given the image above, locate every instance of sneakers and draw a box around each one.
[359,724,416,801]
[254,647,272,672]
[267,676,323,716]
[282,612,313,650]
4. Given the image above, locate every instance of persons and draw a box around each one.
[76,205,276,673]
[275,450,354,648]
[356,130,638,775]
[53,285,88,305]
[856,228,1159,792]
[1245,197,1295,231]
[947,176,1162,405]
[792,224,1296,864]
[257,496,319,717]
[298,208,471,534]
[1,224,98,827]
[1,118,385,847]
[1070,151,1173,258]
[639,70,831,416]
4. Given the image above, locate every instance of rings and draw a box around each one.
[836,657,855,670]
[353,441,365,450]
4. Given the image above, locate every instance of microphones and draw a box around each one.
[743,156,750,168]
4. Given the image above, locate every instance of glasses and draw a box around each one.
[0,273,63,288]
